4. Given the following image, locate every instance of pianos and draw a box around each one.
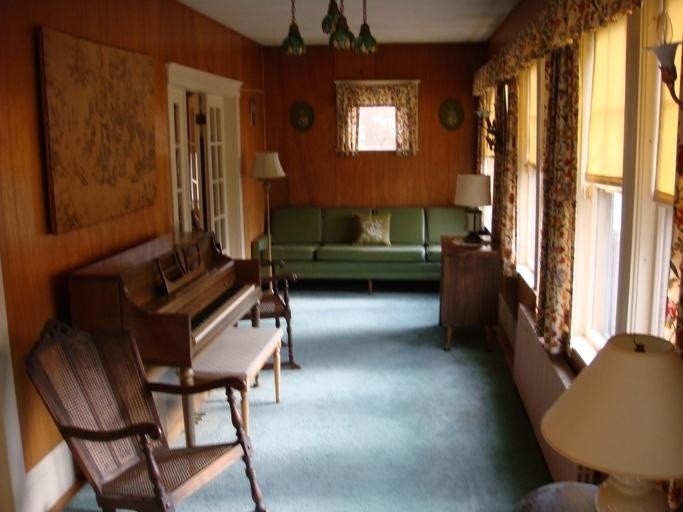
[65,231,262,475]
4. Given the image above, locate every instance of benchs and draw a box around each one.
[189,327,284,439]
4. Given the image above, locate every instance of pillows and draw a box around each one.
[349,208,393,247]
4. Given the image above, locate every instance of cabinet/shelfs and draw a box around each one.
[440,235,500,352]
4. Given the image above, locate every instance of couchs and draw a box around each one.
[252,202,466,293]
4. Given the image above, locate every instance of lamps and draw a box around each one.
[282,1,375,57]
[248,151,287,316]
[454,175,493,244]
[541,333,683,512]
[649,38,683,110]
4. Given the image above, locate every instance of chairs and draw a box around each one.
[24,314,266,511]
